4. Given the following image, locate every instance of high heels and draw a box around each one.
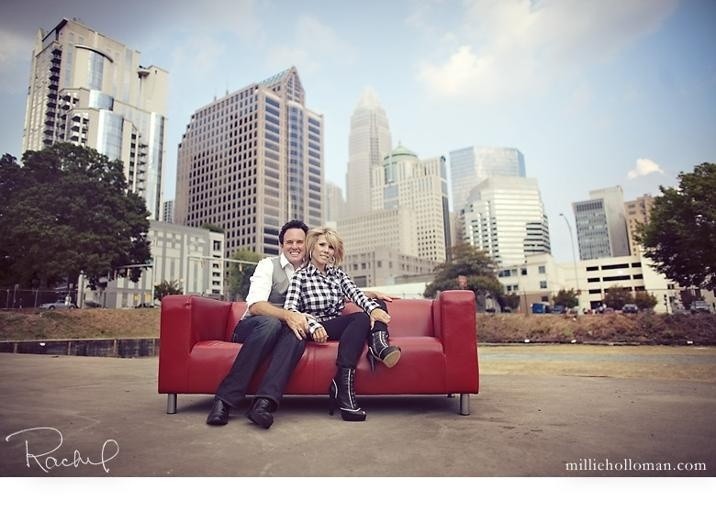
[328,368,366,420]
[366,331,401,372]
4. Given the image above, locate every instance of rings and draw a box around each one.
[299,329,303,333]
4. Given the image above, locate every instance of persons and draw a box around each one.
[288,225,401,421]
[15,296,24,312]
[64,293,72,309]
[205,220,401,429]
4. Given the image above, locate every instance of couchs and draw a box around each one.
[157,289,478,416]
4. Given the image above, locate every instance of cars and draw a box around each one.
[689,300,709,311]
[39,299,76,310]
[533,302,551,313]
[85,299,102,308]
[622,303,639,314]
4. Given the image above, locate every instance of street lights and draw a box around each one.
[558,213,581,309]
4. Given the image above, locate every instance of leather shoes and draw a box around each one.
[245,398,274,429]
[207,400,230,425]
